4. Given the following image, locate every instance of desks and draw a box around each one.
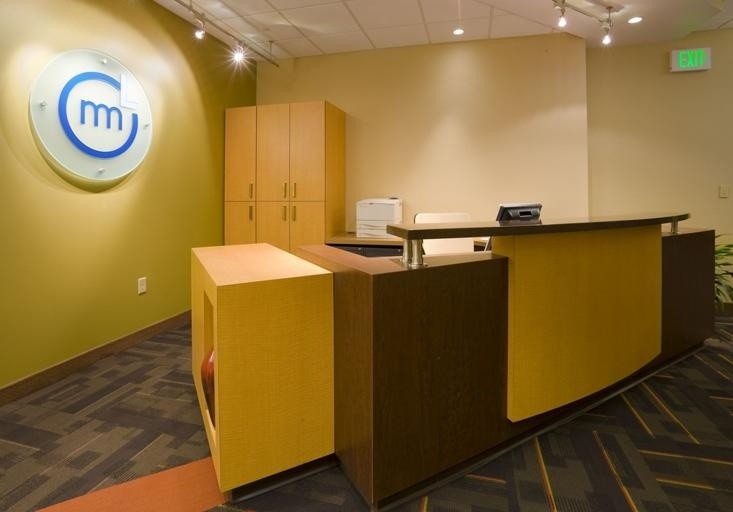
[327,232,405,257]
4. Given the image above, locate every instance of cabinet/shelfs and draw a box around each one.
[223,99,347,257]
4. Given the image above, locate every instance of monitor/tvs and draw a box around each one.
[483,203,543,251]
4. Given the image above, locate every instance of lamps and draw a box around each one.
[553,0,612,45]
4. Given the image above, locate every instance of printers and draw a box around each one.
[356,199,403,240]
